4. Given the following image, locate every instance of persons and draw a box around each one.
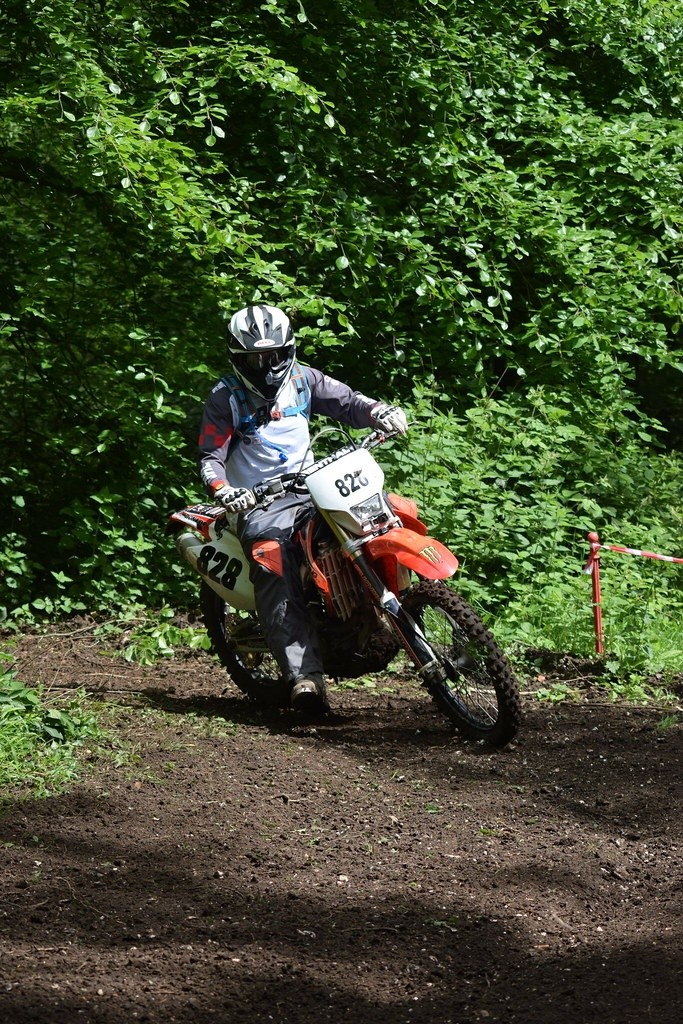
[200,304,410,715]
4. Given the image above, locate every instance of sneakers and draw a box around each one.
[291,673,326,705]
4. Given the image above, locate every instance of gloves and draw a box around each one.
[365,401,408,437]
[209,480,256,514]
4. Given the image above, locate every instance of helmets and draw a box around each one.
[227,305,296,404]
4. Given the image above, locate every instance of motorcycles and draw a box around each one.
[164,433,524,749]
